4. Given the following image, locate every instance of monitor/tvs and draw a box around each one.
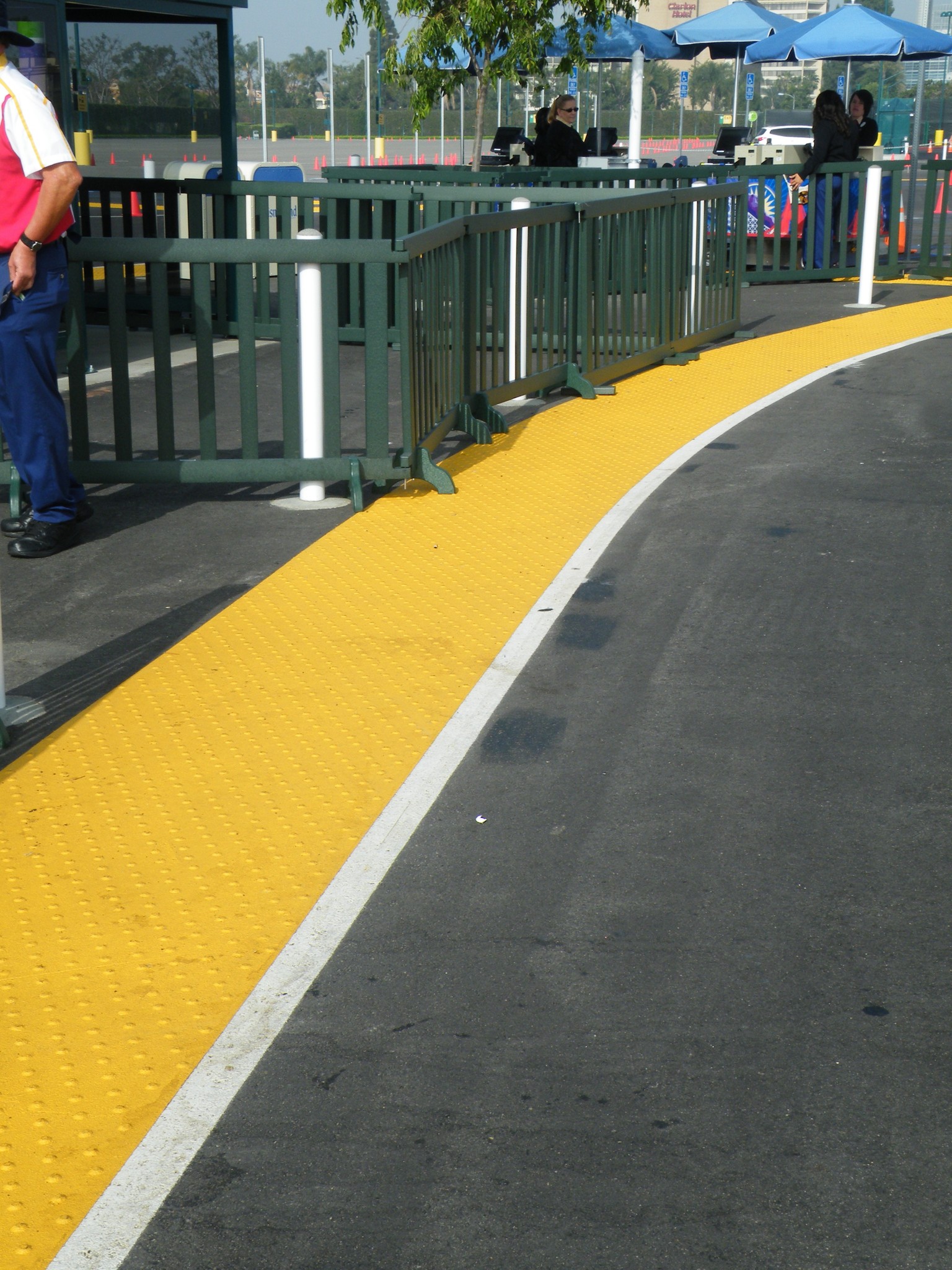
[490,127,525,155]
[583,127,617,151]
[713,127,753,158]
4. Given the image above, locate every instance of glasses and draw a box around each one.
[561,108,578,112]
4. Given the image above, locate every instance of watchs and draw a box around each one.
[19,232,42,253]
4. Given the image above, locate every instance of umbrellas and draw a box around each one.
[376,12,528,165]
[744,3,952,114]
[660,1,801,127]
[535,7,693,157]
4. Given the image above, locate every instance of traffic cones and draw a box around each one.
[337,136,366,142]
[428,137,431,142]
[310,136,313,141]
[110,152,115,165]
[271,155,277,162]
[121,191,143,218]
[386,137,415,143]
[149,153,152,160]
[90,153,96,166]
[883,134,952,255]
[313,157,321,171]
[293,155,297,162]
[347,153,459,166]
[454,137,457,141]
[183,154,206,163]
[433,137,436,142]
[322,155,327,167]
[446,137,449,142]
[292,136,295,141]
[140,154,146,167]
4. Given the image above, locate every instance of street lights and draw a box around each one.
[777,92,796,111]
[188,83,198,143]
[323,93,331,141]
[270,89,277,143]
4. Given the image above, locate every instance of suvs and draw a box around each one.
[748,125,815,148]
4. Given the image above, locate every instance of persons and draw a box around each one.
[518,94,590,167]
[848,89,878,146]
[0,12,91,557]
[789,90,859,269]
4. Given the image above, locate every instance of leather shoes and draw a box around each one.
[7,518,81,558]
[0,504,38,538]
